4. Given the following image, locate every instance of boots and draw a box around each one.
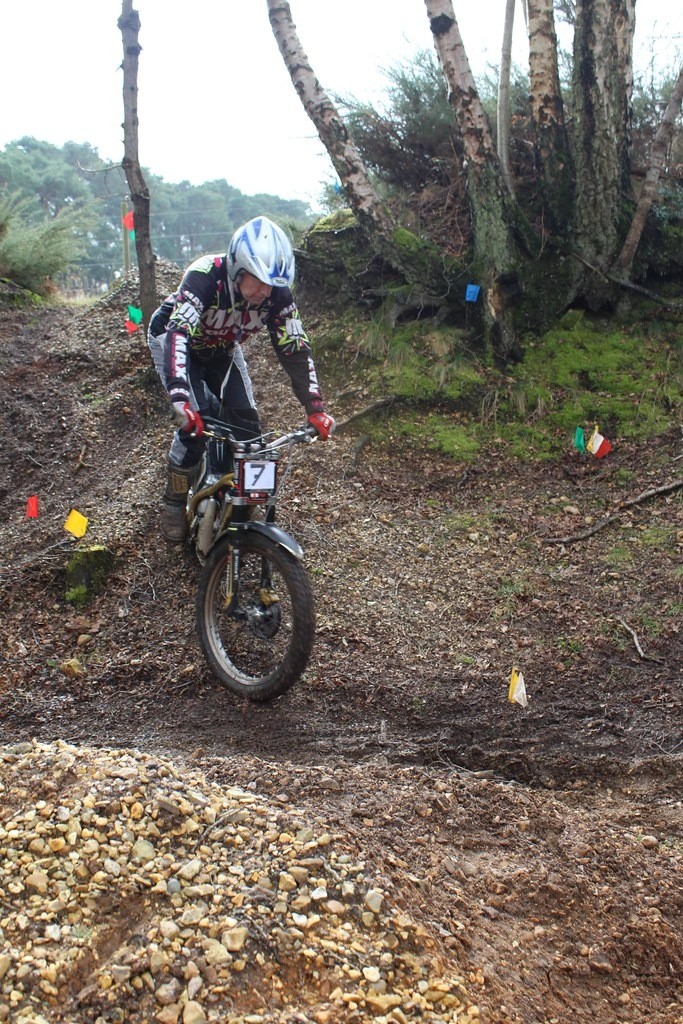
[159,446,202,542]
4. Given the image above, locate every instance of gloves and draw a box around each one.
[168,383,203,442]
[303,399,336,441]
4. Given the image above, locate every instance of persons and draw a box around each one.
[147,215,337,543]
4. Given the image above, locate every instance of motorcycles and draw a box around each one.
[169,409,320,705]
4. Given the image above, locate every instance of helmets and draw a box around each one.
[227,215,295,288]
